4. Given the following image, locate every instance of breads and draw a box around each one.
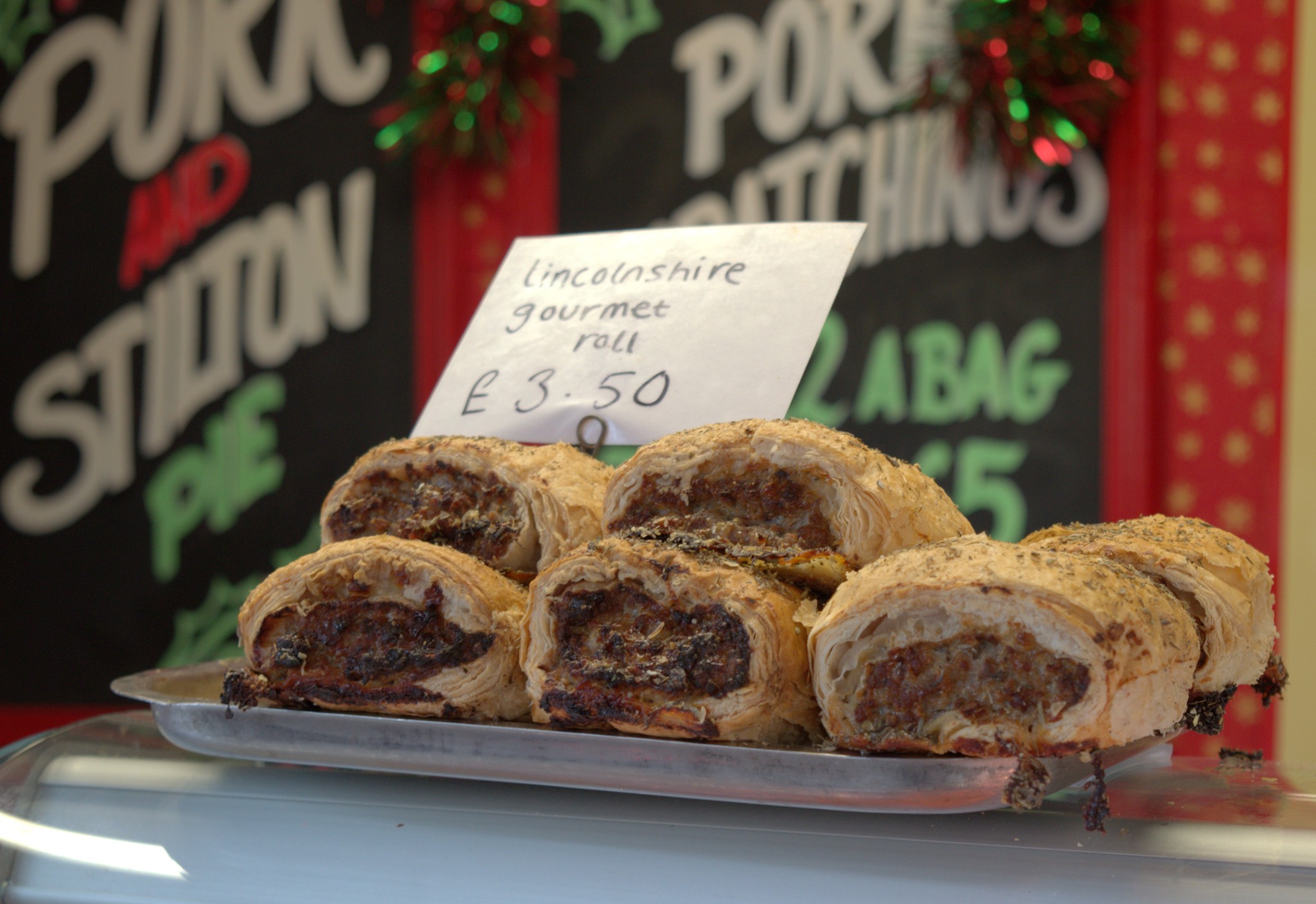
[229,415,1284,760]
